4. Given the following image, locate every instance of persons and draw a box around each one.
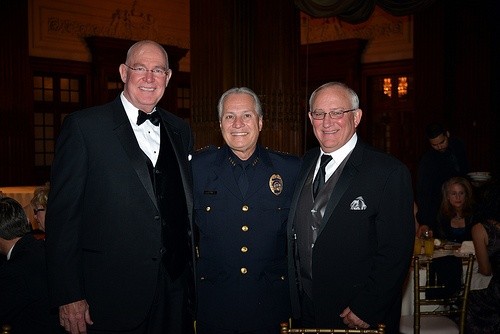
[416,129,500,334]
[286,81,416,334]
[191,87,301,334]
[0,197,59,334]
[47,40,197,334]
[32,186,50,230]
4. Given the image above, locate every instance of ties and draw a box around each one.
[313,154,333,201]
[238,160,250,200]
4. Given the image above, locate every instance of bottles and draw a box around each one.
[423,230,434,259]
[413,235,422,256]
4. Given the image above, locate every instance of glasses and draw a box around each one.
[123,62,168,78]
[33,208,45,215]
[310,108,358,120]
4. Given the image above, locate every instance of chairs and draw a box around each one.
[399,253,476,334]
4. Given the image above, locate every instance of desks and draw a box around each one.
[401,236,493,316]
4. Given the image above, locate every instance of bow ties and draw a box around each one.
[136,110,160,126]
